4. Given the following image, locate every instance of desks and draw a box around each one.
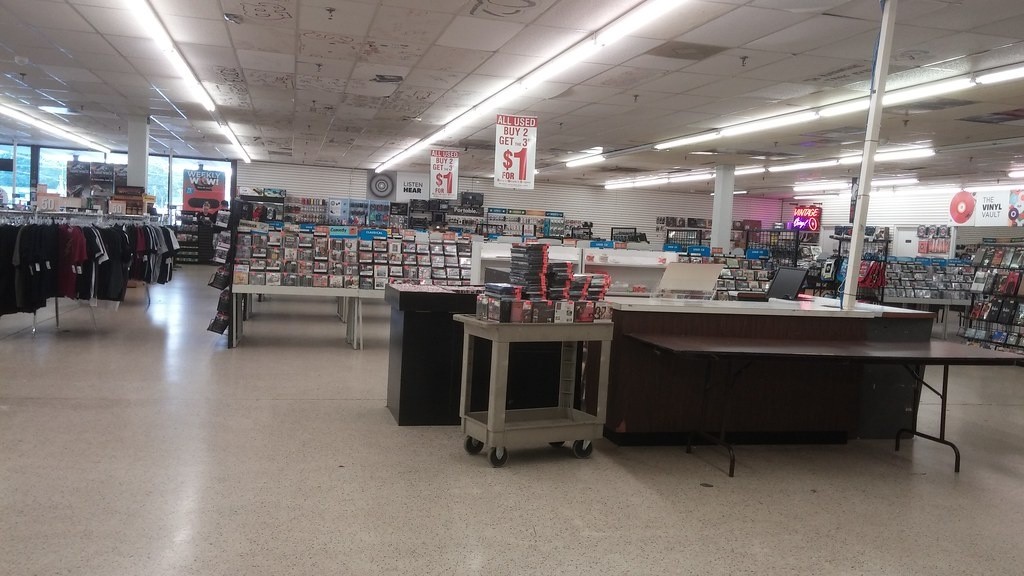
[622,333,1024,473]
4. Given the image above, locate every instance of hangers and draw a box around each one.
[0,210,163,229]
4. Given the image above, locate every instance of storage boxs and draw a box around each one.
[475,295,613,323]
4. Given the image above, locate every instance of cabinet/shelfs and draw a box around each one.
[471,242,679,298]
[176,211,212,263]
[799,230,820,245]
[409,199,449,230]
[454,314,614,467]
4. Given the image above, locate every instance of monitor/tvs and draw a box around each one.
[765,266,809,301]
